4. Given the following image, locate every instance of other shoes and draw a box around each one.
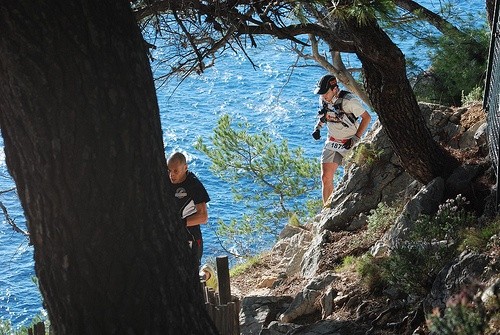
[200,264,217,291]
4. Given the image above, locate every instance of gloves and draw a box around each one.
[343,134,361,150]
[312,125,321,140]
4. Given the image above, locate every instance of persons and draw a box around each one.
[167,152,210,268]
[312,74,371,203]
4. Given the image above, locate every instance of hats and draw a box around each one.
[313,75,337,95]
